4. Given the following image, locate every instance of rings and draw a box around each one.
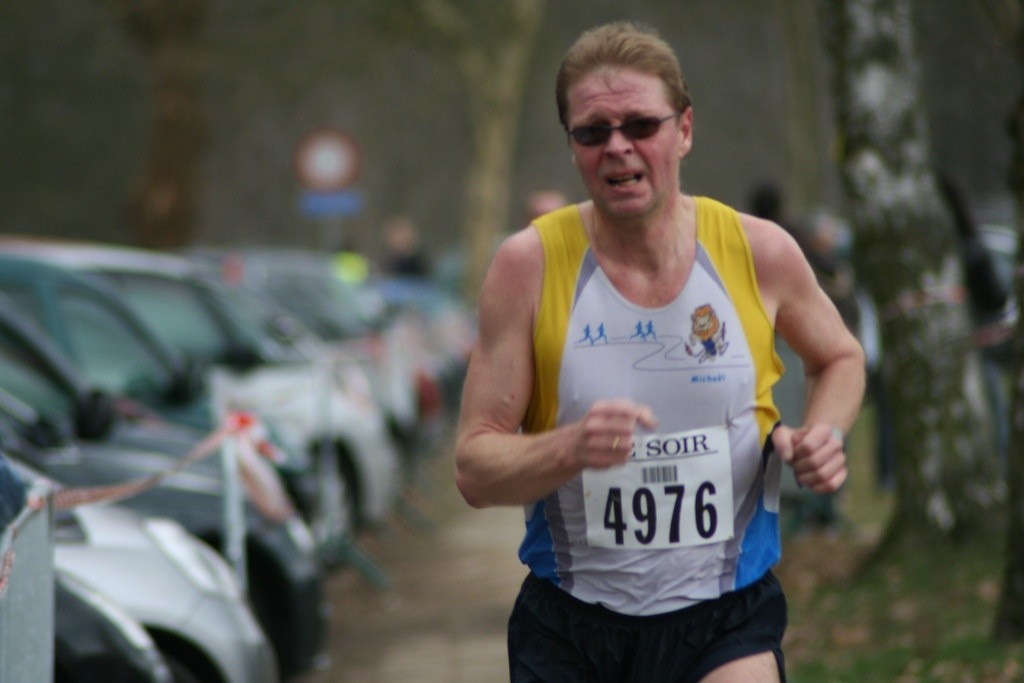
[610,434,620,454]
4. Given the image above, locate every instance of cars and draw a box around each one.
[0,236,471,683]
[975,224,1022,301]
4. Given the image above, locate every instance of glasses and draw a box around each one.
[568,113,678,146]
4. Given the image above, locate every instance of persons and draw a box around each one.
[453,24,866,683]
[383,172,1017,510]
